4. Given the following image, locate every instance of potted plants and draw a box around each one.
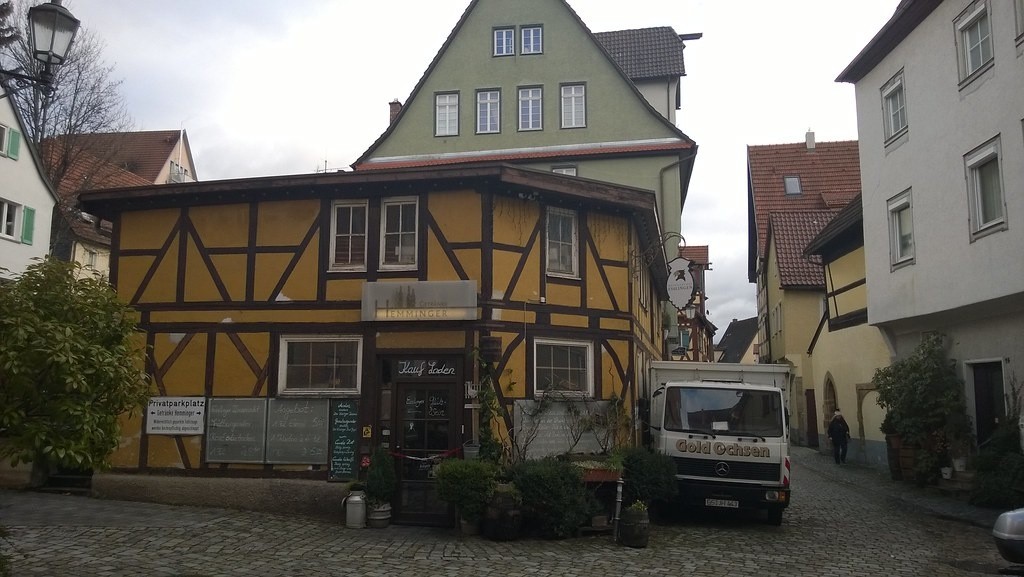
[569,453,624,483]
[433,460,498,535]
[366,474,397,527]
[484,480,524,541]
[618,499,650,549]
[895,413,954,484]
[879,408,902,480]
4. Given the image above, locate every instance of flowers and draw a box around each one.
[360,455,371,473]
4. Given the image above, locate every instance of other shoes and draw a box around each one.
[842,461,847,465]
[835,463,841,467]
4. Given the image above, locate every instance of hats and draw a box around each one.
[834,411,842,416]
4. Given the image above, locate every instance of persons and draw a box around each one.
[828,408,850,466]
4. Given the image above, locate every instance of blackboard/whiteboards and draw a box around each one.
[265,397,330,465]
[327,397,359,482]
[204,396,267,465]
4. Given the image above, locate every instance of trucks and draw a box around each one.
[646,353,794,524]
